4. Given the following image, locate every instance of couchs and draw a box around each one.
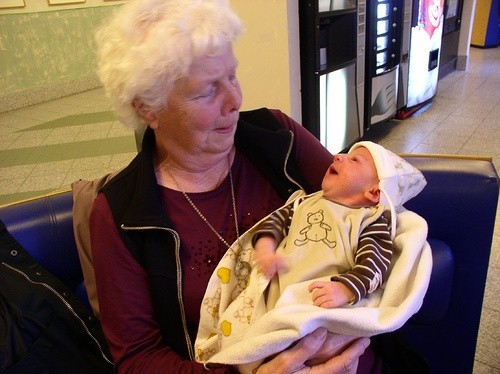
[0,151,500,374]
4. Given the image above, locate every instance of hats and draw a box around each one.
[350,140,427,209]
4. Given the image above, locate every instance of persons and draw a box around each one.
[87,0,430,374]
[193,141,426,366]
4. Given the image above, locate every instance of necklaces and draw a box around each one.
[161,153,252,280]
